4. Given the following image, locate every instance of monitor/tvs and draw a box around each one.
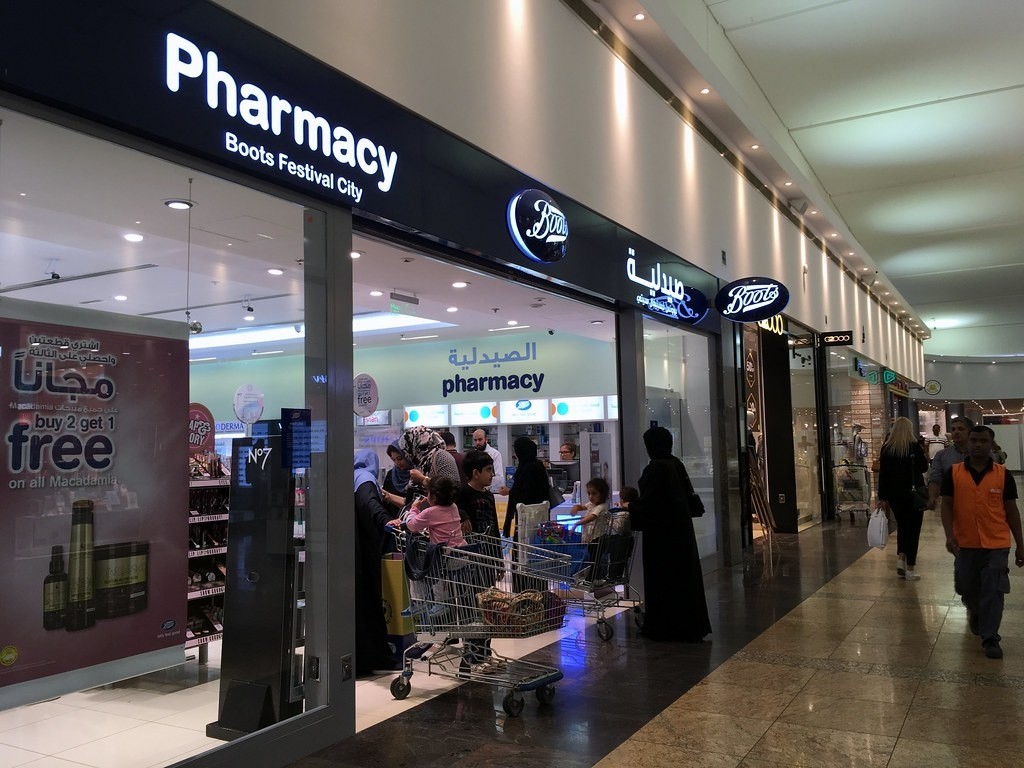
[550,460,580,493]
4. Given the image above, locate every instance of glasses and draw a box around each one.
[559,451,571,455]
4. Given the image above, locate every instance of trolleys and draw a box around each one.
[383,520,573,716]
[494,509,646,642]
[830,464,871,521]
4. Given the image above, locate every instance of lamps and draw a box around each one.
[861,275,875,286]
[899,316,909,324]
[788,199,808,215]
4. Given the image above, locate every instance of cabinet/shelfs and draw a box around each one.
[184,476,231,664]
[460,433,600,466]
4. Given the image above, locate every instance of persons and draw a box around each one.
[938,425,1024,659]
[853,423,866,466]
[623,427,712,640]
[747,411,759,513]
[878,417,929,581]
[882,417,1007,510]
[353,425,637,680]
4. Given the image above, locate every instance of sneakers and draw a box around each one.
[401,602,427,617]
[429,604,448,616]
[897,559,905,576]
[906,571,920,581]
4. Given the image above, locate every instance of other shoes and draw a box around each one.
[486,655,507,670]
[968,610,981,635]
[373,660,408,674]
[471,663,496,674]
[577,576,623,587]
[985,642,1003,658]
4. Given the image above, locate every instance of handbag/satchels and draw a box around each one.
[867,507,888,550]
[690,492,705,518]
[549,486,565,510]
[475,585,544,633]
